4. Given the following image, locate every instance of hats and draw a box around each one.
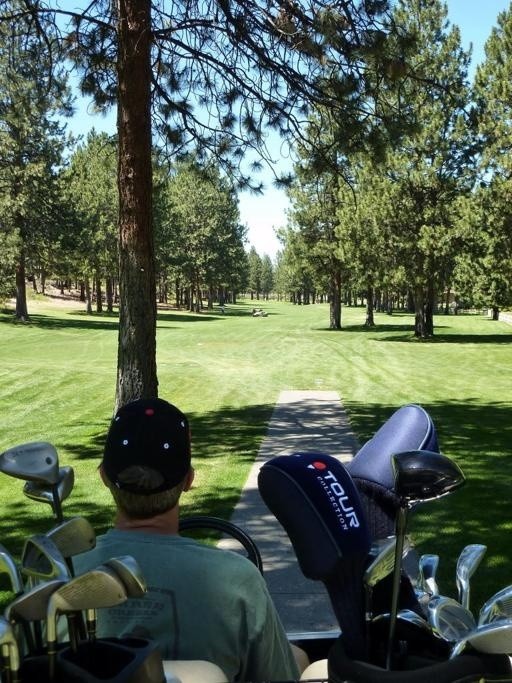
[102,395,191,496]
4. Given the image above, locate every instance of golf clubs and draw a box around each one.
[371,548,512,666]
[0,442,147,683]
[385,451,467,663]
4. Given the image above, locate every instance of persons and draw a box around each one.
[20,395,311,683]
